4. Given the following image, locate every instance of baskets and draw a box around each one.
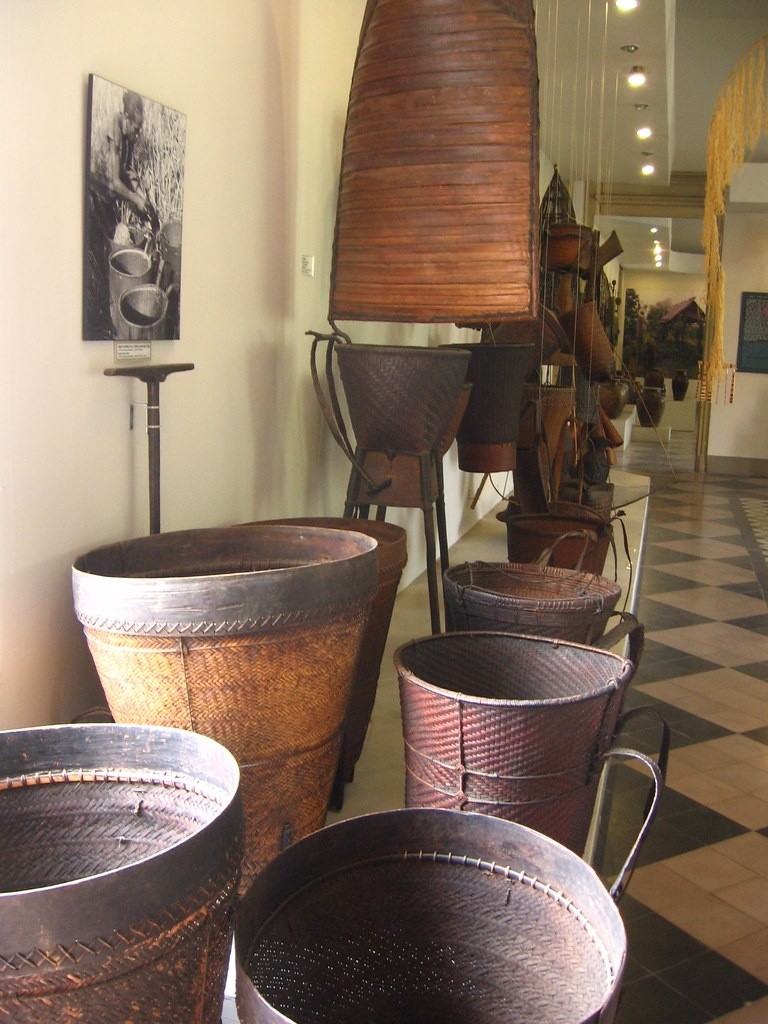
[0,222,670,1024]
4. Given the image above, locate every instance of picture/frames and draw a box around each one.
[736,291,768,374]
